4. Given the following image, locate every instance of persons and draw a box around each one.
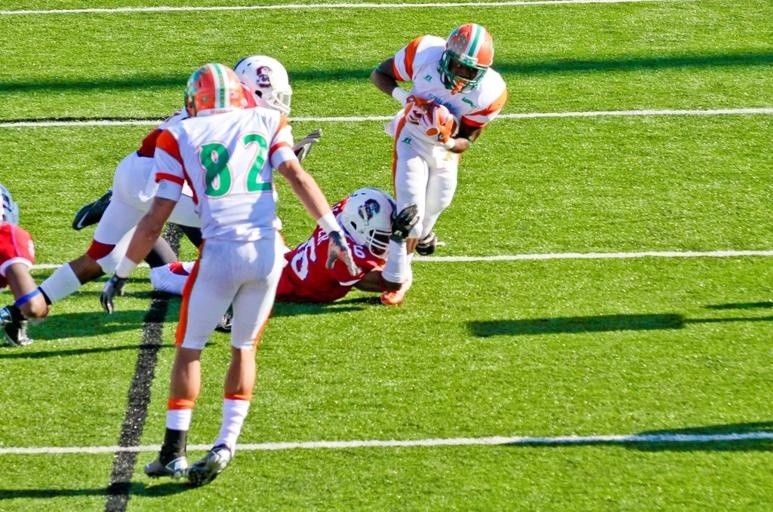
[72,127,322,250]
[99,63,360,488]
[0,179,50,320]
[0,56,293,346]
[368,22,508,305]
[150,185,420,302]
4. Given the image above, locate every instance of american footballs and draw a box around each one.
[419,104,457,137]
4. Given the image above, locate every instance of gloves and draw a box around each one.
[323,230,363,279]
[98,274,127,315]
[290,128,322,163]
[389,202,420,243]
[402,93,435,126]
[417,104,456,146]
[415,231,440,256]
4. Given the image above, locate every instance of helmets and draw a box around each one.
[0,183,20,225]
[336,184,398,251]
[182,62,244,117]
[444,23,495,83]
[229,54,291,110]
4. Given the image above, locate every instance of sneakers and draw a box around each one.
[144,456,189,478]
[0,304,35,346]
[69,188,114,231]
[188,444,233,489]
[381,276,415,307]
